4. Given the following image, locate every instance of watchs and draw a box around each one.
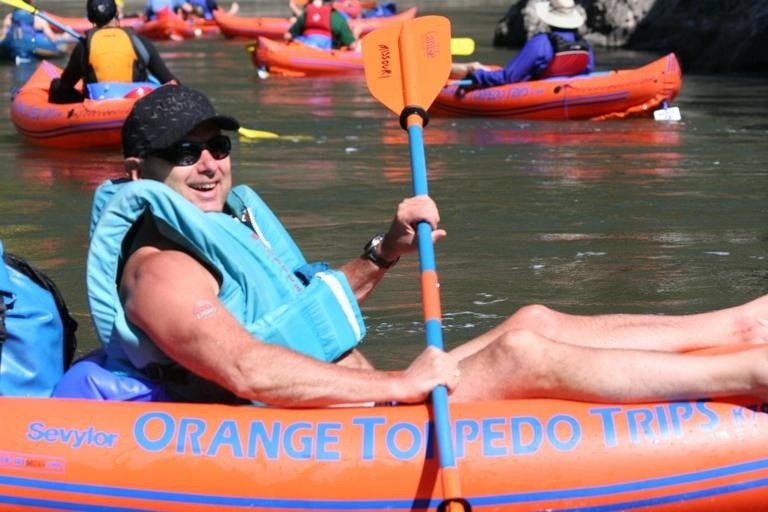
[364,233,400,269]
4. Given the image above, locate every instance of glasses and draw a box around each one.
[157,136,232,167]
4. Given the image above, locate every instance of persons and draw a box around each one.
[86,84,767,411]
[0,1,172,106]
[450,0,592,106]
[148,1,396,53]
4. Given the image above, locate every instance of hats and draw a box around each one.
[86,0,116,23]
[123,84,240,159]
[537,1,588,30]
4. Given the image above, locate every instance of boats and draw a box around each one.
[9,59,178,149]
[131,4,220,40]
[0,394,768,512]
[0,19,68,63]
[211,5,418,40]
[249,34,365,75]
[38,2,240,37]
[426,51,682,121]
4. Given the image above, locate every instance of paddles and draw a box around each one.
[361,16,468,512]
[146,73,280,139]
[1,0,85,40]
